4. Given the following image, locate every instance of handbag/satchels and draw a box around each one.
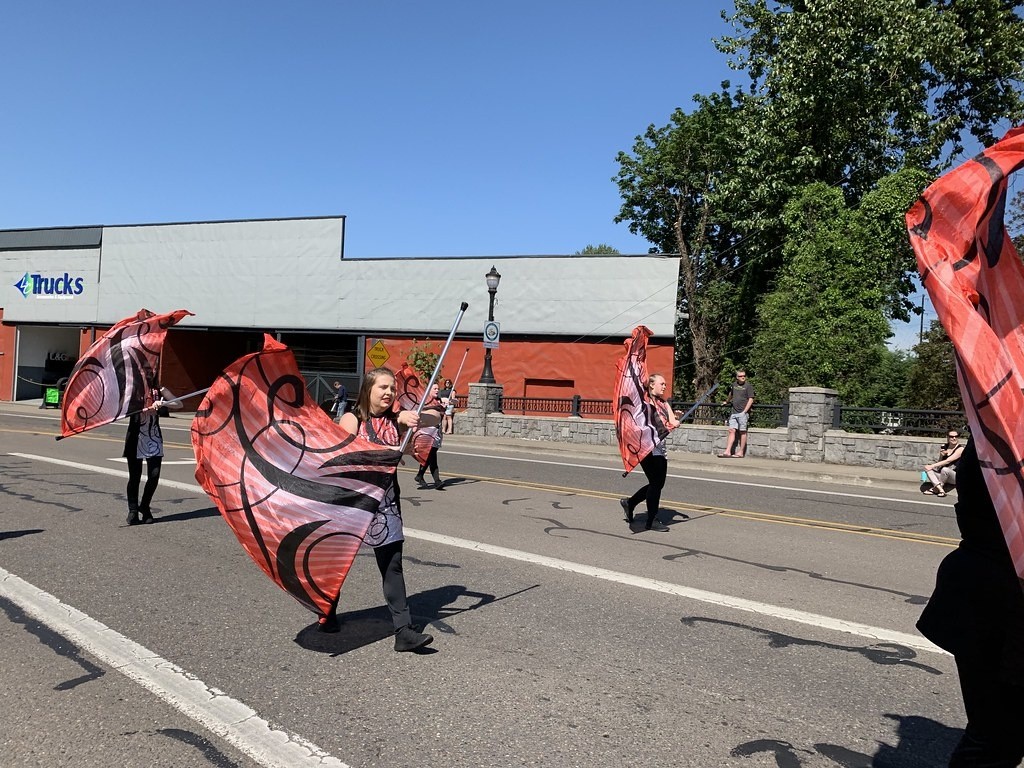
[331,401,338,411]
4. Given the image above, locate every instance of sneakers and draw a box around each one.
[619,499,635,524]
[393,627,436,652]
[645,515,670,532]
[317,617,341,633]
[139,504,154,524]
[126,510,139,526]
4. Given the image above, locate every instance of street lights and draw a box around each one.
[478,265,501,383]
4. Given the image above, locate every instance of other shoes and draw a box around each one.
[717,453,731,458]
[732,453,744,458]
[415,476,428,489]
[433,481,447,490]
[444,432,453,434]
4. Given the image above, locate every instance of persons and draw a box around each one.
[333,381,348,417]
[415,383,447,490]
[717,369,755,458]
[917,427,1024,768]
[439,379,457,434]
[122,385,183,525]
[620,374,681,532]
[923,430,965,498]
[319,367,432,651]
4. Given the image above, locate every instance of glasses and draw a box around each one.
[737,375,746,377]
[445,382,450,383]
[948,435,958,438]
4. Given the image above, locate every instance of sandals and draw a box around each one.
[925,487,946,497]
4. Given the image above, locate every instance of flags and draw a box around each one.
[61,308,195,437]
[612,326,676,473]
[905,124,1024,580]
[390,364,446,466]
[192,334,401,621]
